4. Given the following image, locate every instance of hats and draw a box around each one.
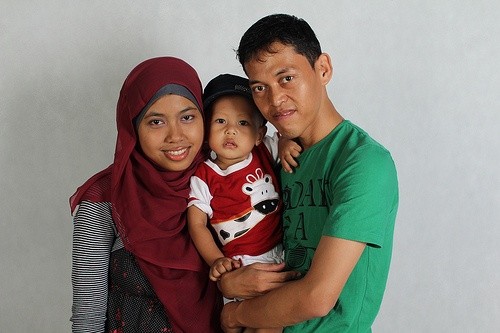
[202,73,268,126]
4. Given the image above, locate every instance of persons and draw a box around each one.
[67,56,226,333]
[215,13,399,333]
[186,72,303,332]
